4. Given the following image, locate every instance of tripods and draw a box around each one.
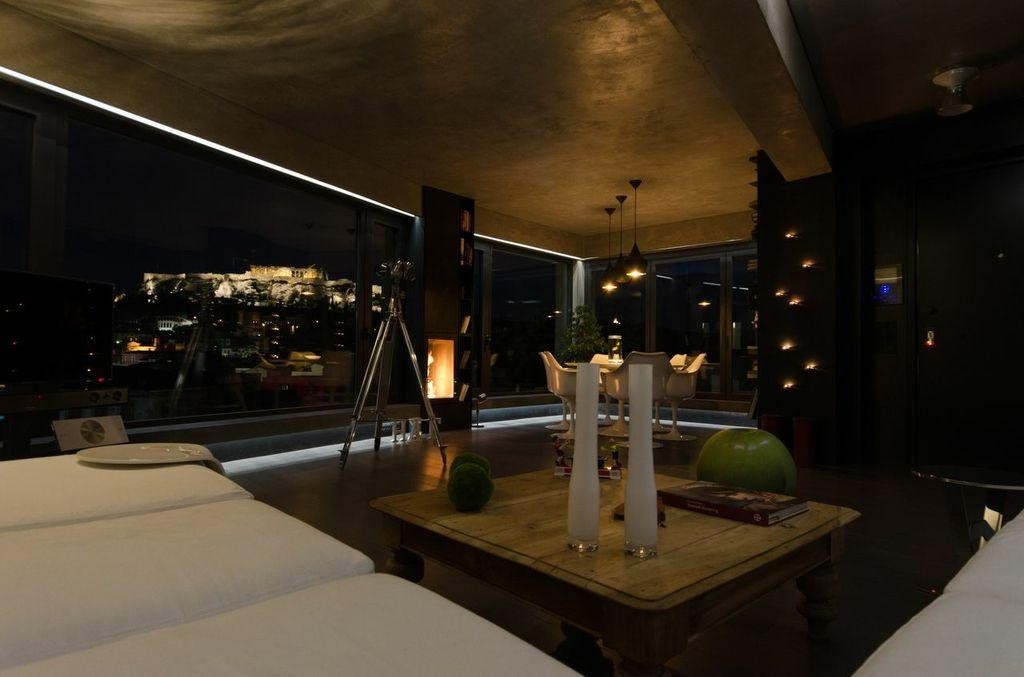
[340,290,447,471]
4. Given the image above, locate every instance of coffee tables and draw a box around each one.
[370,467,860,677]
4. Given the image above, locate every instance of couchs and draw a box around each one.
[0,455,581,677]
[851,507,1024,677]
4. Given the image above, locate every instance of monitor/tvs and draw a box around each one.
[0,267,115,385]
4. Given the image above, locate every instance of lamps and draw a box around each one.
[927,62,979,117]
[623,180,647,279]
[600,208,621,294]
[610,195,631,284]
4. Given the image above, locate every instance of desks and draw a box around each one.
[565,362,686,436]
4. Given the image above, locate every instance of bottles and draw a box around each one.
[565,364,601,555]
[624,365,657,559]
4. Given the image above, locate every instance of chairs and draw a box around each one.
[538,351,707,441]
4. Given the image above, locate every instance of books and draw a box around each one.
[657,480,810,527]
